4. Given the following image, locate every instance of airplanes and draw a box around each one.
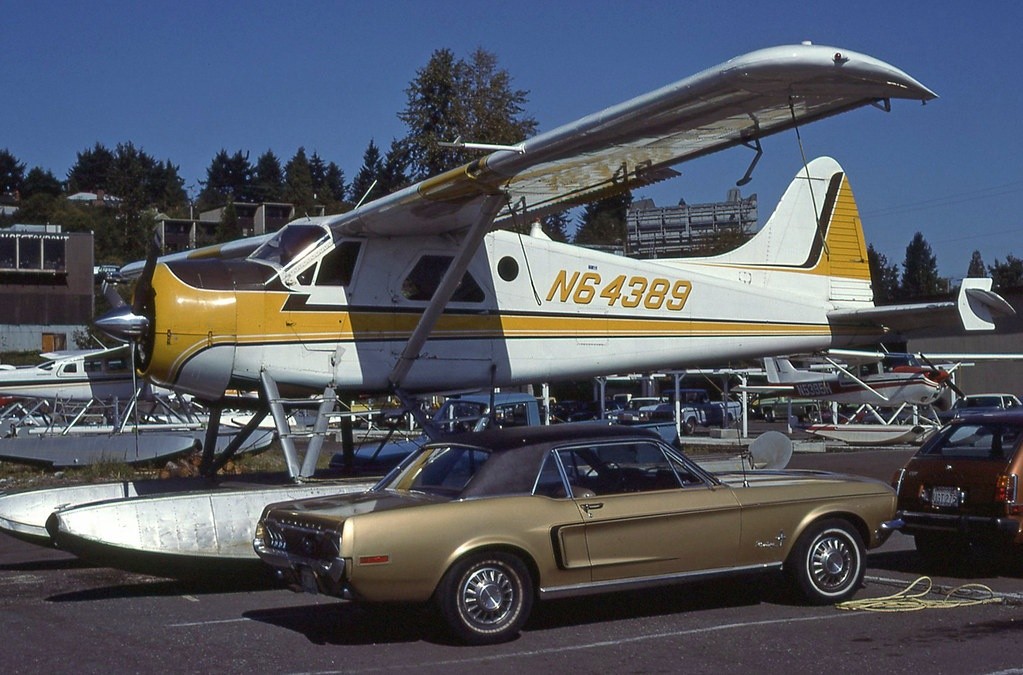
[0,342,184,400]
[92,40,1017,405]
[730,352,967,404]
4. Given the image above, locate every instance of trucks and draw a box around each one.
[328,392,683,483]
[638,389,741,435]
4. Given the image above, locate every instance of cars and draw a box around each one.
[572,400,625,421]
[941,394,1023,411]
[253,422,898,645]
[606,398,669,420]
[890,410,1023,566]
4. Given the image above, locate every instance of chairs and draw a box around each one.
[550,484,595,498]
